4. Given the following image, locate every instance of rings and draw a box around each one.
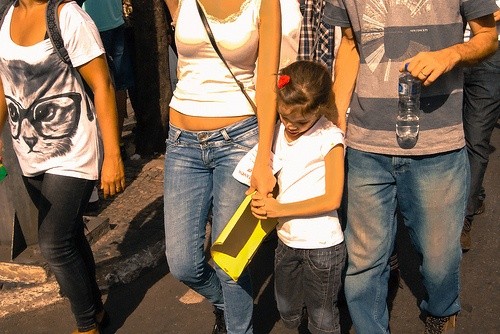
[420,71,428,76]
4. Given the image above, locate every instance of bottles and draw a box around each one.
[395,62,423,138]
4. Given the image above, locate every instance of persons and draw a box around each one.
[164,0,282,334]
[0,0,126,334]
[321,0,500,334]
[0,0,500,270]
[249,61,348,334]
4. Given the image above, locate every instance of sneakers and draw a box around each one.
[211,306,228,334]
[424,313,456,334]
[71,310,108,334]
[460,202,486,250]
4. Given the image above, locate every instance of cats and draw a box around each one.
[0,51,77,165]
